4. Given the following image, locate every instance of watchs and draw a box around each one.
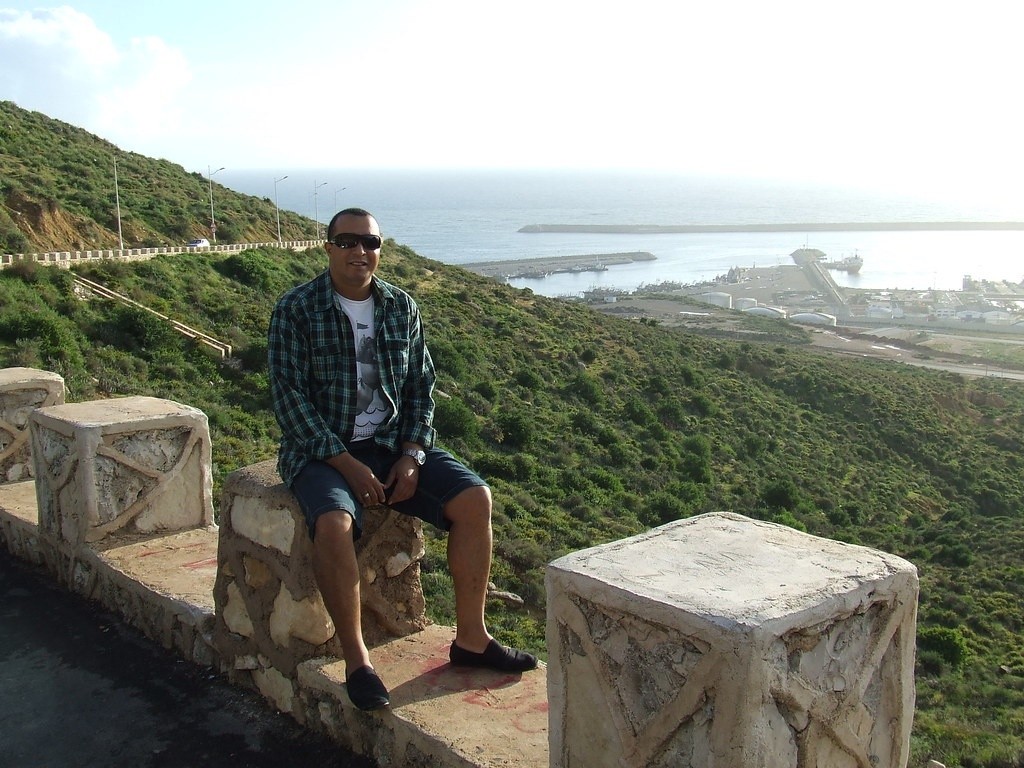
[401,448,426,467]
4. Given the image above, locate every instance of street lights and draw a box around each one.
[210,165,224,245]
[335,185,346,213]
[313,179,327,241]
[274,174,288,245]
[112,155,127,250]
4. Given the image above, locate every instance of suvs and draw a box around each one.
[187,239,209,247]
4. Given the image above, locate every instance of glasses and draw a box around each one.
[330,233,382,250]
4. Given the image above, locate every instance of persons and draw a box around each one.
[267,207,539,712]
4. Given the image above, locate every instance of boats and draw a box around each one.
[837,249,866,272]
[493,258,609,278]
[583,264,746,296]
[790,240,826,262]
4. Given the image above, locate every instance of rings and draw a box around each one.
[362,492,369,499]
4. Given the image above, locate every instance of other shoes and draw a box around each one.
[345,663,390,711]
[449,637,538,672]
